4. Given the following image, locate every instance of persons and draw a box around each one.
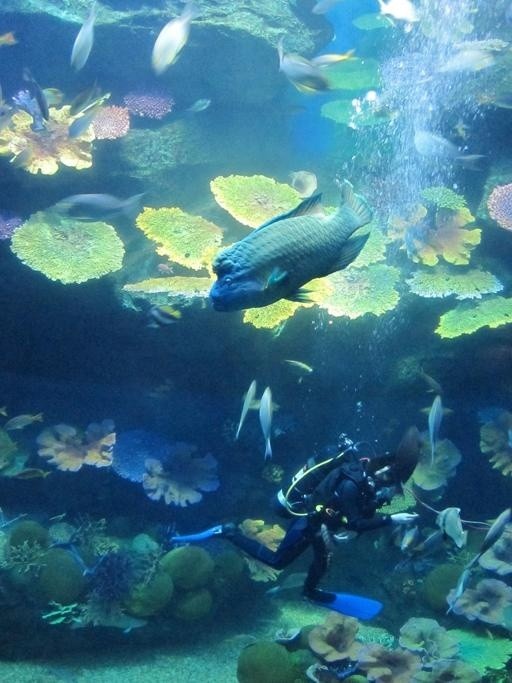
[170,451,421,623]
